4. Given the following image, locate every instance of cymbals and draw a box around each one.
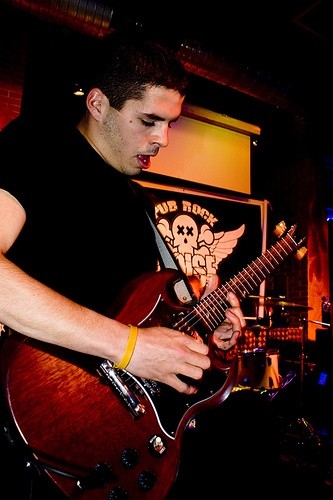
[251,301,314,311]
[248,295,281,300]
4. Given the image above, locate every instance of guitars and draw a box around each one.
[0,219,307,500]
